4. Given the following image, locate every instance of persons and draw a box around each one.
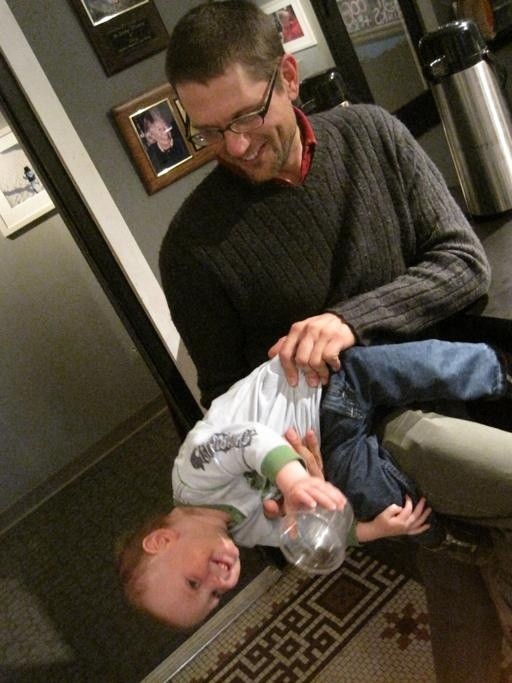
[24,166,39,193]
[153,2,512,518]
[112,341,512,635]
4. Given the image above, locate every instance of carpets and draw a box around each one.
[141,545,511,683]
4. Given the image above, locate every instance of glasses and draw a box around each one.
[183,55,282,148]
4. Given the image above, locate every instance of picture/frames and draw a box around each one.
[70,0,170,78]
[258,0,318,54]
[110,83,218,195]
[0,125,56,239]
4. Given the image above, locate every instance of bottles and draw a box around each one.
[417,18,512,222]
[298,67,364,115]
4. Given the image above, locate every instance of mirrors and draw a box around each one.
[309,0,444,140]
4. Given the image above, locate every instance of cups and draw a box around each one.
[277,501,354,576]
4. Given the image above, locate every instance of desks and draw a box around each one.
[453,216,512,352]
